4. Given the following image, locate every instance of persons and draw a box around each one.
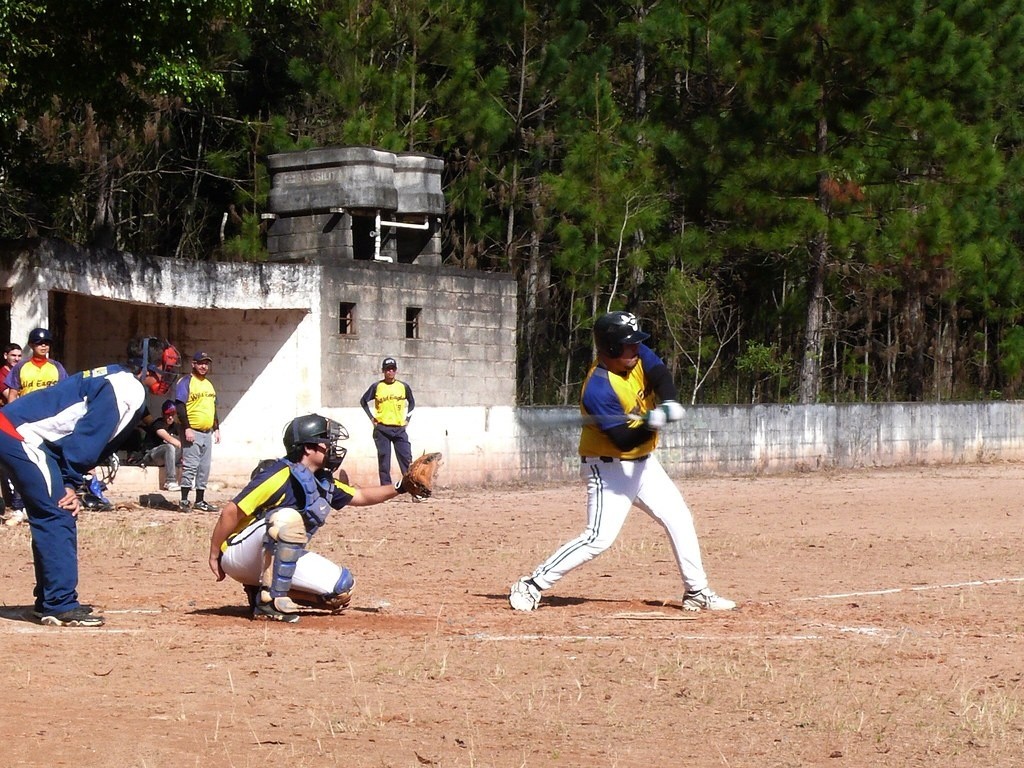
[360,356,429,504]
[0,334,180,626]
[174,350,221,513]
[139,400,184,492]
[209,414,442,622]
[509,310,736,612]
[0,321,155,527]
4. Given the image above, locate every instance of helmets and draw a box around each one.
[283,413,328,455]
[594,310,652,358]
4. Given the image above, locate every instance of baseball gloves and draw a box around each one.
[394,448,445,504]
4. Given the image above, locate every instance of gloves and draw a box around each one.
[643,409,668,431]
[659,399,687,421]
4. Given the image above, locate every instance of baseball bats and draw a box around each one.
[517,414,648,434]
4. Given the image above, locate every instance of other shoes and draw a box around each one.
[125,456,139,466]
[163,481,182,491]
[4,507,30,526]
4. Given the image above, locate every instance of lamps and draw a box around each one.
[330,208,347,214]
[261,213,278,219]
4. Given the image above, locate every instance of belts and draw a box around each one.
[194,429,210,433]
[580,454,649,463]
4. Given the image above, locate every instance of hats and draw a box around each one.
[28,328,53,345]
[382,358,396,369]
[162,400,177,415]
[193,351,213,363]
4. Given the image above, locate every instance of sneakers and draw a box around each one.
[40,607,106,627]
[508,575,542,612]
[243,583,269,616]
[192,501,220,514]
[83,475,110,509]
[682,588,736,611]
[412,494,427,503]
[253,602,299,623]
[176,499,195,513]
[32,604,93,618]
